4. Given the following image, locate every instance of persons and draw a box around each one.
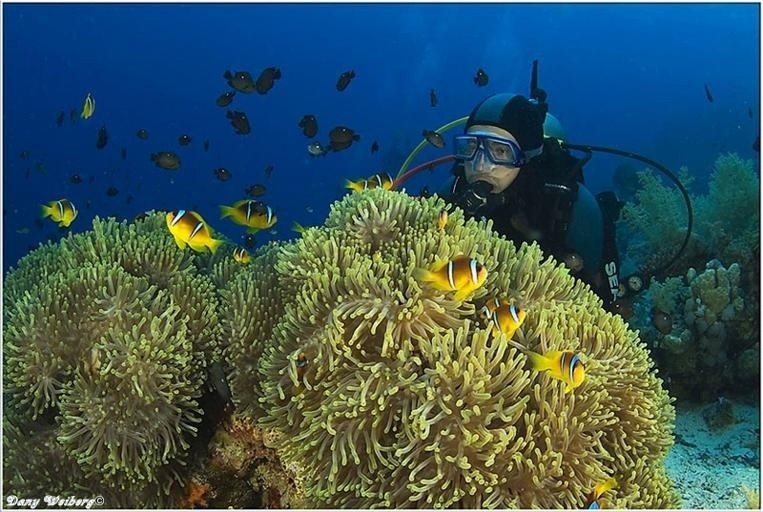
[435,91,607,303]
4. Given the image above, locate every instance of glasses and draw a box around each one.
[451,132,544,168]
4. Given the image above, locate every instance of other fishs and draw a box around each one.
[13,65,489,263]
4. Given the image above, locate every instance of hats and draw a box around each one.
[465,93,543,195]
